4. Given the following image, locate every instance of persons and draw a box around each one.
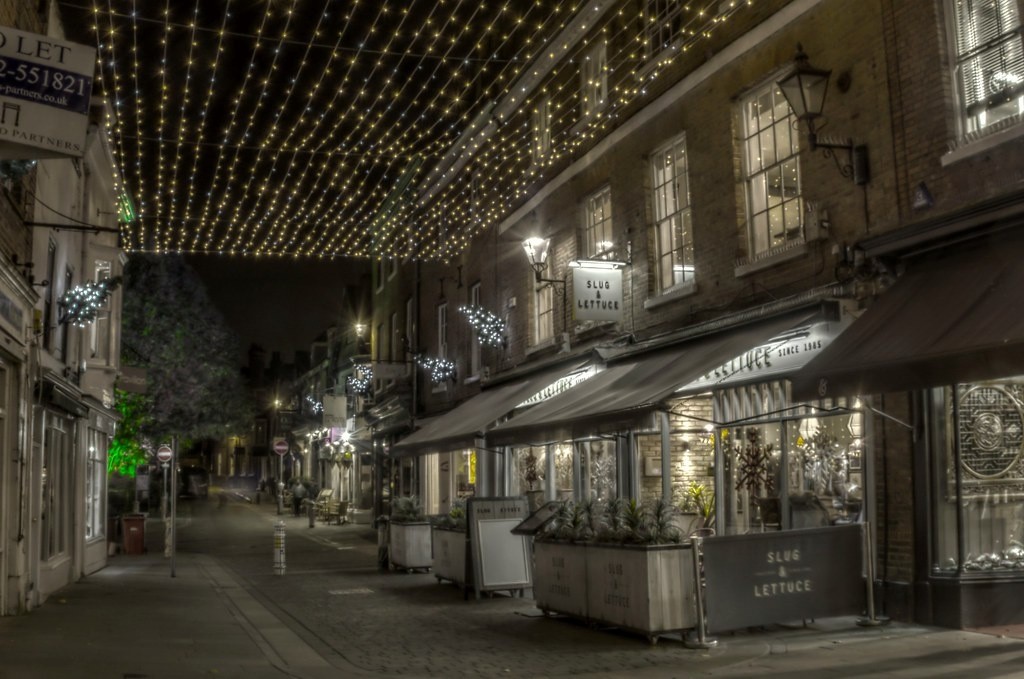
[292,479,307,516]
[304,477,321,528]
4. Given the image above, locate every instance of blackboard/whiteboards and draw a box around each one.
[463,494,531,590]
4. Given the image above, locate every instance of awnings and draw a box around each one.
[486,303,820,450]
[788,228,1024,405]
[395,357,593,454]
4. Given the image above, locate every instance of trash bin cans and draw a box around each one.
[108,514,120,556]
[121,512,146,554]
[277,495,284,515]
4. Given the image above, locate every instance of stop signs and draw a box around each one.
[157,446,172,462]
[273,441,289,455]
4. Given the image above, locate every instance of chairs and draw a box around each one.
[304,488,348,526]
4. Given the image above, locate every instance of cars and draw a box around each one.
[218,474,262,505]
[151,463,209,499]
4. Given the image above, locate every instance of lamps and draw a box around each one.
[520,210,551,283]
[776,42,833,152]
